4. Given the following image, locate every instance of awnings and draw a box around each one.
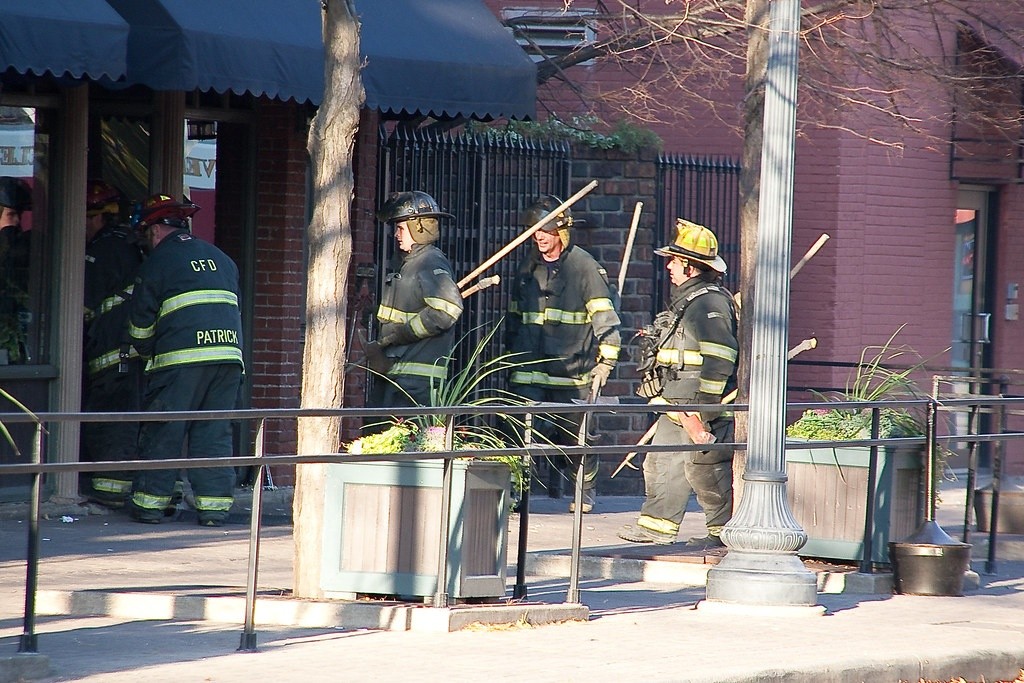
[0,0,537,122]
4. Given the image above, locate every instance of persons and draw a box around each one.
[127,194,245,526]
[79,180,185,516]
[618,218,740,547]
[0,176,32,230]
[361,190,464,433]
[504,194,621,513]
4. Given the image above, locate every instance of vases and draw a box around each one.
[323,452,509,597]
[787,437,927,569]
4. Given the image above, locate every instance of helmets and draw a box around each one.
[86,179,123,211]
[129,194,201,233]
[653,218,727,272]
[517,193,573,232]
[0,176,32,211]
[377,192,457,223]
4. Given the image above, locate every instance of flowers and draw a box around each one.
[346,316,588,534]
[788,320,960,507]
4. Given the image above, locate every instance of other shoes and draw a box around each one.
[199,515,223,526]
[569,501,593,513]
[617,524,677,546]
[138,516,160,524]
[685,534,726,550]
[81,488,124,508]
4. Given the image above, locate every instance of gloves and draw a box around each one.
[590,363,614,387]
[382,332,400,347]
